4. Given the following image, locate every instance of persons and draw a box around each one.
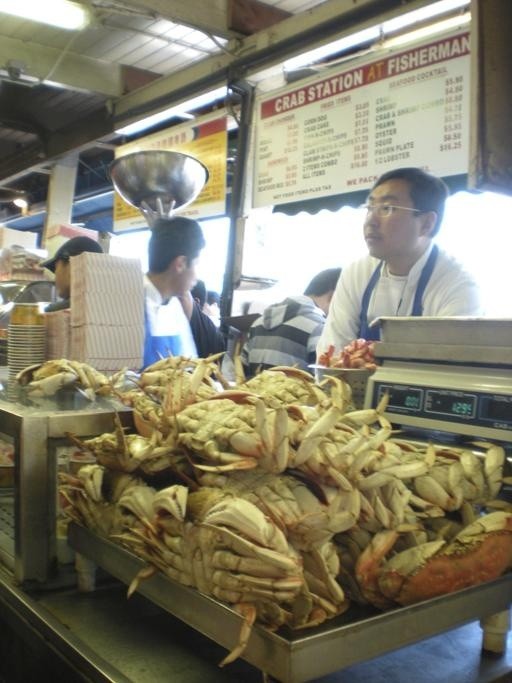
[316,167,476,364]
[39,235,104,312]
[240,268,341,379]
[176,280,223,357]
[138,216,208,369]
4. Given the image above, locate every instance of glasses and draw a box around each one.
[362,201,424,221]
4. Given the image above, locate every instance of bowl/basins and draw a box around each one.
[107,151,209,214]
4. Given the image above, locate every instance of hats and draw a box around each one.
[38,235,105,273]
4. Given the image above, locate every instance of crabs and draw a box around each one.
[57,334,512,669]
[15,357,125,405]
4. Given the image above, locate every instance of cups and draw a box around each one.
[308,364,372,411]
[6,303,46,404]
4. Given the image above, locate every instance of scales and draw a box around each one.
[363,316,512,443]
